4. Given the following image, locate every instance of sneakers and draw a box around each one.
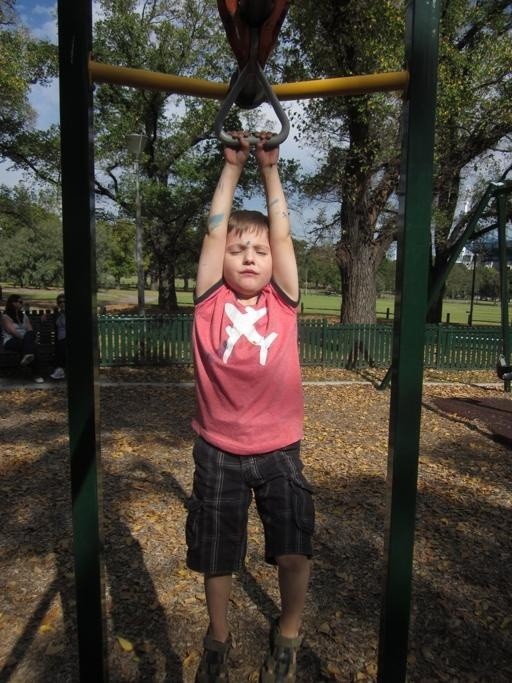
[20,354,65,384]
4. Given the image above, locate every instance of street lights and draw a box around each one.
[467,240,481,327]
[126,128,149,319]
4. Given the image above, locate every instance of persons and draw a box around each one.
[49,294,65,378]
[186,131,316,682]
[0,295,44,383]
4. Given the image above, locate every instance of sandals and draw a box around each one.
[257,616,305,683]
[194,623,233,683]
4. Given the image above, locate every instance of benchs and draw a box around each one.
[0,310,61,367]
